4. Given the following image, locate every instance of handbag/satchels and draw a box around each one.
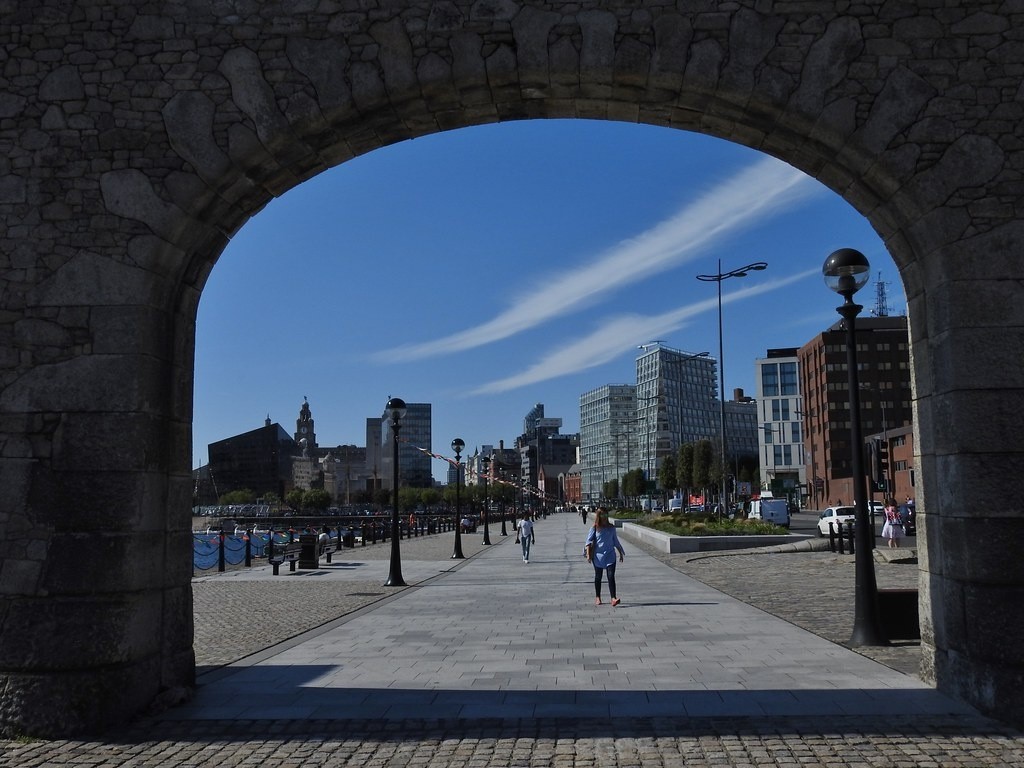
[583,542,595,559]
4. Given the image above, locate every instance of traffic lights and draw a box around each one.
[877,473,886,490]
[871,435,889,484]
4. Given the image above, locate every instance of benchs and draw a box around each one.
[268,544,337,574]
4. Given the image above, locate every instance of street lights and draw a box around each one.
[663,350,709,445]
[697,257,770,522]
[820,248,899,649]
[610,430,635,499]
[381,396,409,588]
[510,473,518,531]
[450,437,466,558]
[634,390,671,514]
[480,455,494,545]
[517,477,557,522]
[498,466,508,536]
[618,415,646,473]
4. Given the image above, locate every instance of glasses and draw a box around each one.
[600,514,608,518]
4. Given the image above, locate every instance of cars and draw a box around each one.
[882,503,917,536]
[651,507,663,514]
[816,505,855,539]
[867,500,885,516]
[672,504,715,513]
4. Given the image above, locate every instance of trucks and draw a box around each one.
[747,499,791,531]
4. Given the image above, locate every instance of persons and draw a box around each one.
[460,517,472,528]
[882,498,902,548]
[578,506,588,524]
[517,513,535,563]
[318,526,331,541]
[585,507,625,606]
[542,507,548,519]
[829,498,842,506]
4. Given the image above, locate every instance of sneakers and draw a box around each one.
[525,560,530,564]
[612,598,620,607]
[595,597,601,605]
